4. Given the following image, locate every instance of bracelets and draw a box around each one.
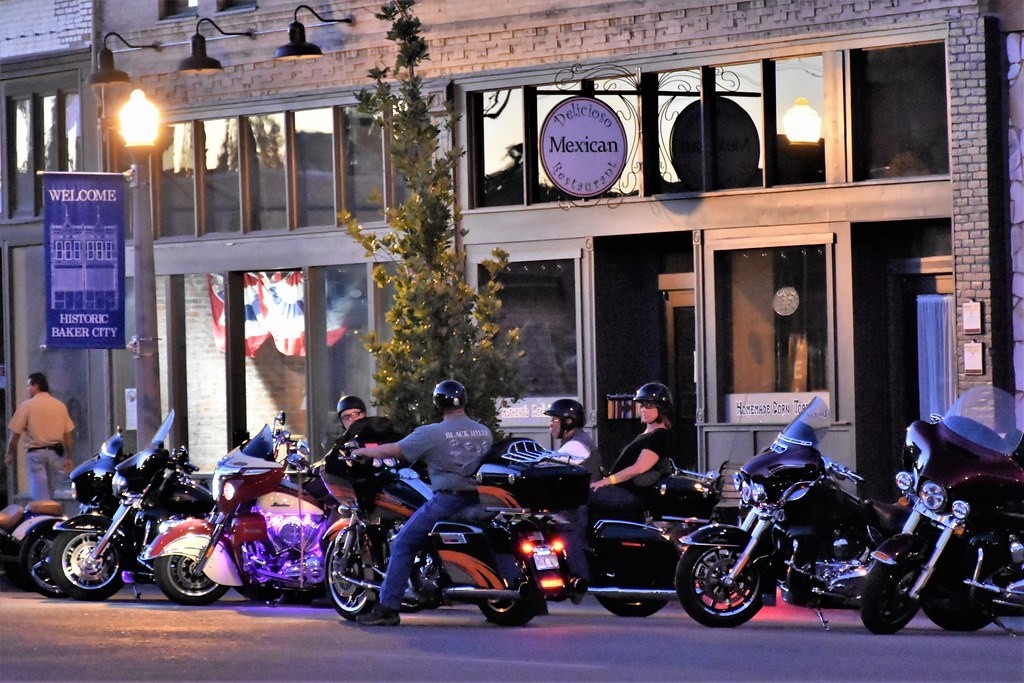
[609,475,616,484]
[4,452,13,457]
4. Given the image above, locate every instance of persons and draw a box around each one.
[349,377,494,625]
[589,380,679,492]
[4,372,75,503]
[535,399,607,582]
[307,394,383,468]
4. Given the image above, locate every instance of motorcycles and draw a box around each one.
[858,383,1024,638]
[0,406,724,619]
[674,395,1000,635]
[318,416,567,627]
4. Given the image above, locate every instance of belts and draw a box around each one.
[26,445,54,452]
[438,488,479,497]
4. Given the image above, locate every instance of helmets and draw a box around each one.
[433,380,467,411]
[543,399,587,433]
[337,395,366,416]
[632,383,673,410]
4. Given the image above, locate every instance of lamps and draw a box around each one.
[272,5,353,63]
[175,17,255,76]
[86,33,161,88]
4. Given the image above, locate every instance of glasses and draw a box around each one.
[639,401,659,409]
[549,415,562,422]
[340,410,362,421]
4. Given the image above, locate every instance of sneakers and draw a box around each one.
[356,606,400,625]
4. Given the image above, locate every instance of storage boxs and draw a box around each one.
[593,521,662,579]
